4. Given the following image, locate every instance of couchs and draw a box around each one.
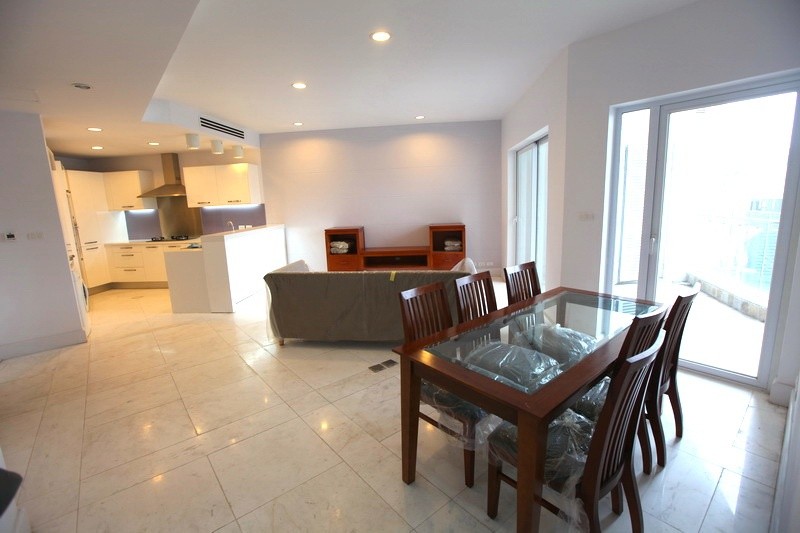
[262,257,477,346]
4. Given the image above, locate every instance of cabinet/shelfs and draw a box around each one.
[65,163,263,289]
[324,223,466,271]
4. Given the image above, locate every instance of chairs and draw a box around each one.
[399,260,701,533]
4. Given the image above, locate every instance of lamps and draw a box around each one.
[186,133,199,149]
[212,140,223,154]
[232,144,243,159]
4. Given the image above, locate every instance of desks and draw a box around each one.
[392,287,671,533]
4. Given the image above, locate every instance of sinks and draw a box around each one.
[182,244,201,249]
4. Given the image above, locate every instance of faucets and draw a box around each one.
[227,221,235,230]
